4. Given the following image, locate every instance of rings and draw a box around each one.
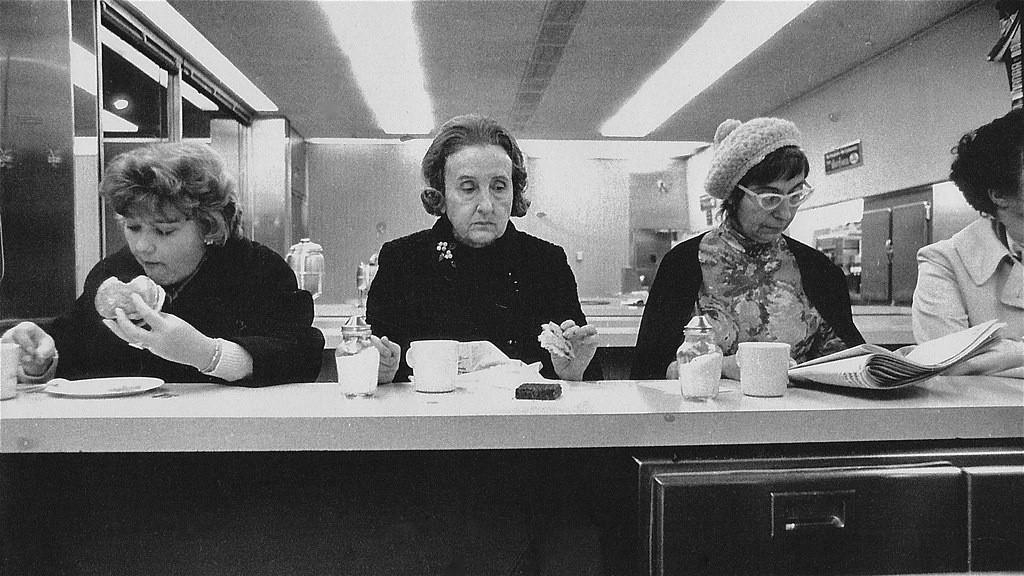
[128,342,143,350]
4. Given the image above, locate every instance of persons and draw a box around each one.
[912,108,1024,375]
[0,139,325,388]
[363,114,605,381]
[630,117,867,381]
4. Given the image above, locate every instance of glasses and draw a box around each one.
[736,180,815,211]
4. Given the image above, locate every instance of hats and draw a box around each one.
[704,117,802,199]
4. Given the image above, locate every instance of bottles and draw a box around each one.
[335,316,380,399]
[676,317,722,400]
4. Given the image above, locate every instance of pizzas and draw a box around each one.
[537,321,575,361]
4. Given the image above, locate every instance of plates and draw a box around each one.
[44,376,163,396]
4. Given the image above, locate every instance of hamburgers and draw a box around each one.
[95,274,166,325]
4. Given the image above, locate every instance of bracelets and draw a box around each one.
[198,338,219,372]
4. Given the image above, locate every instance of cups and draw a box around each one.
[738,342,789,396]
[0,344,20,400]
[406,340,459,393]
[357,265,378,307]
